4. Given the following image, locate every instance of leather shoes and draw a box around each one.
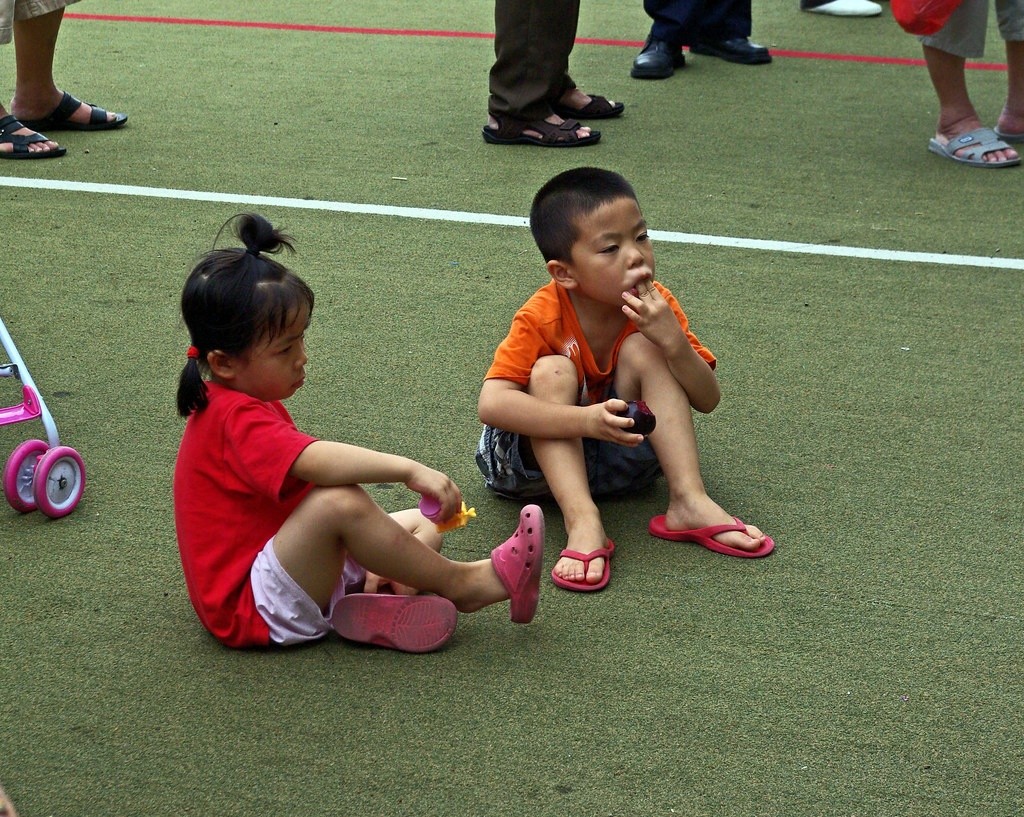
[630,30,682,81]
[689,33,772,65]
[803,0,883,17]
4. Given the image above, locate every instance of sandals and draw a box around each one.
[0,91,128,160]
[481,82,625,148]
[491,504,544,624]
[332,594,458,652]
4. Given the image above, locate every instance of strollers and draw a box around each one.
[0,314,87,516]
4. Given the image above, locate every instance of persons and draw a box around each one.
[174,213,546,654]
[631,1,773,80]
[479,1,625,147]
[892,0,1024,169]
[802,0,882,18]
[474,168,775,595]
[0,1,127,161]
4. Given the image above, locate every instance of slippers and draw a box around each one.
[928,126,1024,168]
[648,514,774,559]
[551,537,616,593]
